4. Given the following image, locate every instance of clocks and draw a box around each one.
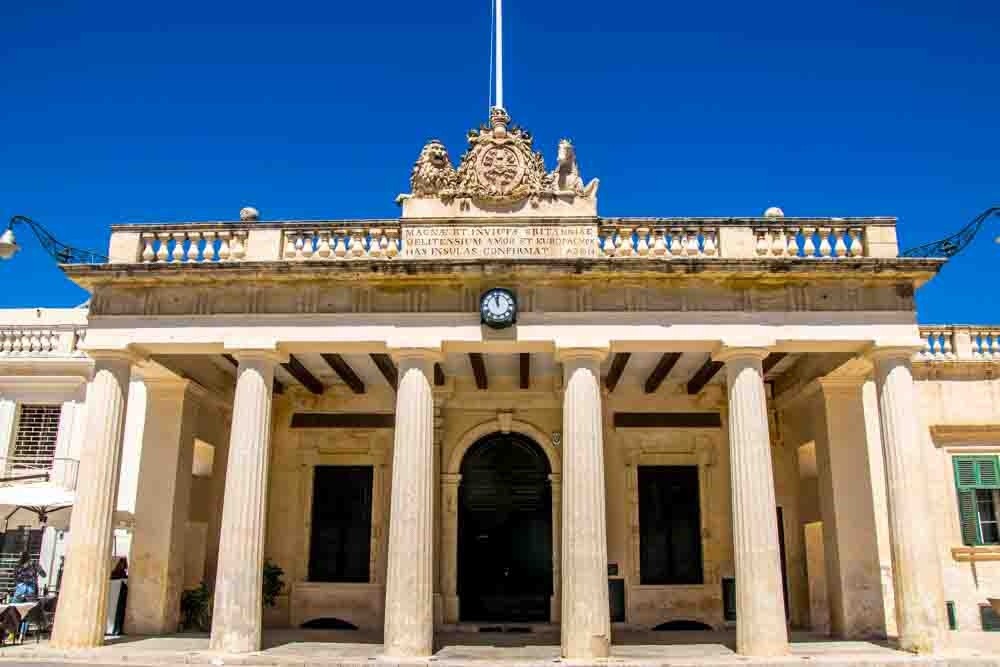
[479,288,517,329]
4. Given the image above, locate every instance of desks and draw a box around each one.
[0,601,41,644]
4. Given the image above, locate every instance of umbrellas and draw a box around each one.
[0,483,76,546]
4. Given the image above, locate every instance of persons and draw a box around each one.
[8,551,47,641]
[105,556,129,634]
[56,555,64,599]
